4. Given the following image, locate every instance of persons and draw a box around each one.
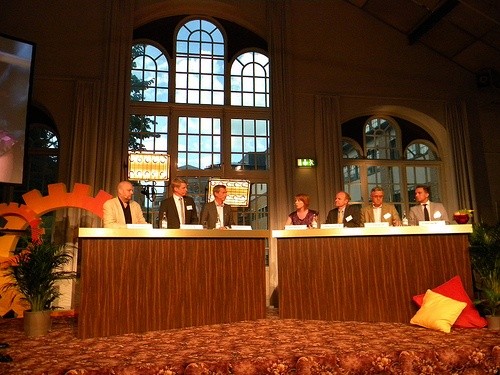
[284,193,319,229]
[360,186,402,226]
[200,184,236,230]
[0,73,21,184]
[324,191,360,227]
[102,181,149,228]
[158,179,199,229]
[405,185,449,226]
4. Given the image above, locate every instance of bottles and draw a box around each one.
[312,214,318,228]
[402,210,408,226]
[216,216,221,229]
[161,211,168,229]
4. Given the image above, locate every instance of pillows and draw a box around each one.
[411,275,488,329]
[409,289,467,334]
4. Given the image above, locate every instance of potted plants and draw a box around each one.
[468,217,500,330]
[0,233,82,337]
[453,208,474,224]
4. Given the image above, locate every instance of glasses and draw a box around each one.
[371,194,382,198]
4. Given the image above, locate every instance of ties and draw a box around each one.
[179,197,184,223]
[423,203,429,220]
